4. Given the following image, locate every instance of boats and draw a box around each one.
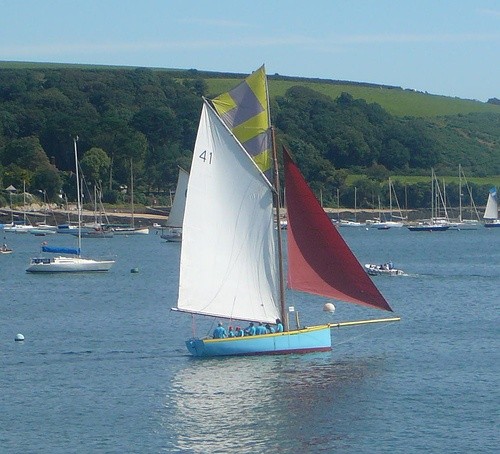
[1,249,13,255]
[364,263,408,277]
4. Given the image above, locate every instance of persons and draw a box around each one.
[212,321,227,339]
[244,322,256,335]
[274,319,283,332]
[255,322,267,335]
[369,260,393,271]
[234,326,243,337]
[265,325,276,333]
[228,326,235,337]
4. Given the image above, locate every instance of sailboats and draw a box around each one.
[445,162,483,229]
[171,64,401,357]
[24,136,115,272]
[408,168,451,231]
[341,186,366,226]
[56,176,95,233]
[30,189,58,230]
[71,179,114,238]
[3,185,39,232]
[155,164,189,243]
[371,177,404,228]
[482,185,500,227]
[114,156,149,235]
